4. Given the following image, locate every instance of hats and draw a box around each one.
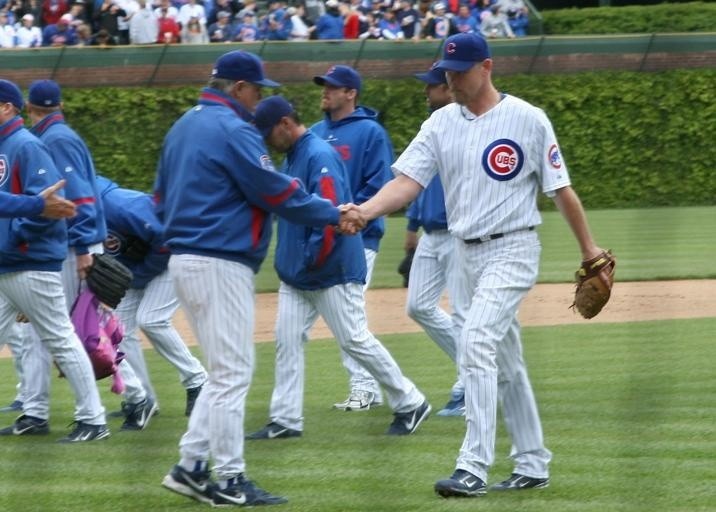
[218,10,254,18]
[434,3,446,12]
[434,34,492,71]
[412,60,447,85]
[211,50,281,88]
[22,11,82,26]
[2,79,23,109]
[325,0,339,9]
[253,96,293,138]
[313,65,360,89]
[28,80,61,106]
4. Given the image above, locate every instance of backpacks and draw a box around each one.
[54,287,127,382]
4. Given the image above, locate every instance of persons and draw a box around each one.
[92,177,211,416]
[337,32,618,499]
[305,63,393,414]
[246,95,435,444]
[1,0,534,47]
[396,62,469,419]
[153,51,366,509]
[0,81,154,443]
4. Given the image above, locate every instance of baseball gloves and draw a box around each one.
[85,253,133,309]
[572,248,616,320]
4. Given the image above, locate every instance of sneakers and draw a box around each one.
[437,391,469,417]
[335,393,385,412]
[434,470,490,498]
[494,471,550,492]
[109,400,159,431]
[1,415,49,434]
[246,422,303,440]
[162,462,290,508]
[389,402,432,436]
[185,380,204,416]
[59,423,112,443]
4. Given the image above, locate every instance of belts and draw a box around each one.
[464,233,506,245]
